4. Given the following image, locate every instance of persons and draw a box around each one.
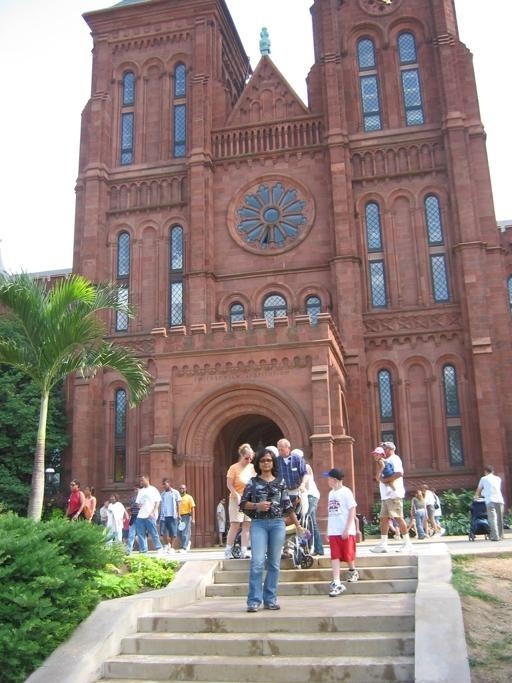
[475,464,505,542]
[386,484,446,542]
[371,446,398,493]
[241,449,306,613]
[367,440,413,555]
[66,474,195,553]
[216,438,324,560]
[321,467,360,597]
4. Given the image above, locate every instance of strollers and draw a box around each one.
[232,518,254,555]
[468,496,494,542]
[284,508,314,568]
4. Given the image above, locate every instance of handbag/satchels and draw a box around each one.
[242,509,256,517]
[82,506,91,519]
[434,504,439,510]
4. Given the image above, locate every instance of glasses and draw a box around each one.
[259,458,273,463]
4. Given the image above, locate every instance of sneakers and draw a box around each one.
[264,604,280,610]
[369,545,388,553]
[396,544,413,552]
[347,570,360,581]
[393,528,446,541]
[329,582,346,597]
[247,604,258,611]
[224,549,325,561]
[156,540,192,554]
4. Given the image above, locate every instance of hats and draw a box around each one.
[369,447,387,458]
[321,469,344,480]
